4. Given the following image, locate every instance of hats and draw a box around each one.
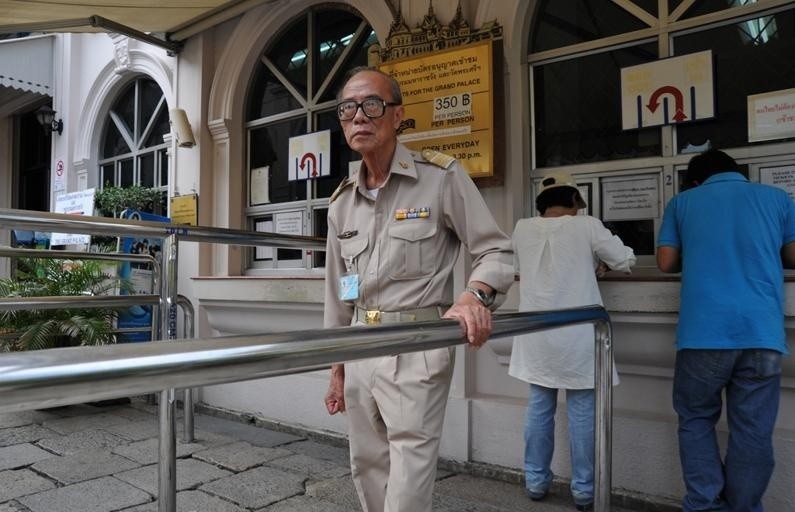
[534,172,587,208]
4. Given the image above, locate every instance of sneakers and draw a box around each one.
[575,500,593,510]
[526,486,544,499]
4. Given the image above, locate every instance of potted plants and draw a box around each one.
[0,233,138,412]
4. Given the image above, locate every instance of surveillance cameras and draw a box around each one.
[167,108,196,149]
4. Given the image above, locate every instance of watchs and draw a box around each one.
[466,285,490,310]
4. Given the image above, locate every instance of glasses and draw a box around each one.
[336,97,397,121]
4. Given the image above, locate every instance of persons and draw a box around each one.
[655,149,795,511]
[131,213,140,220]
[131,238,161,271]
[511,171,637,512]
[324,66,518,511]
